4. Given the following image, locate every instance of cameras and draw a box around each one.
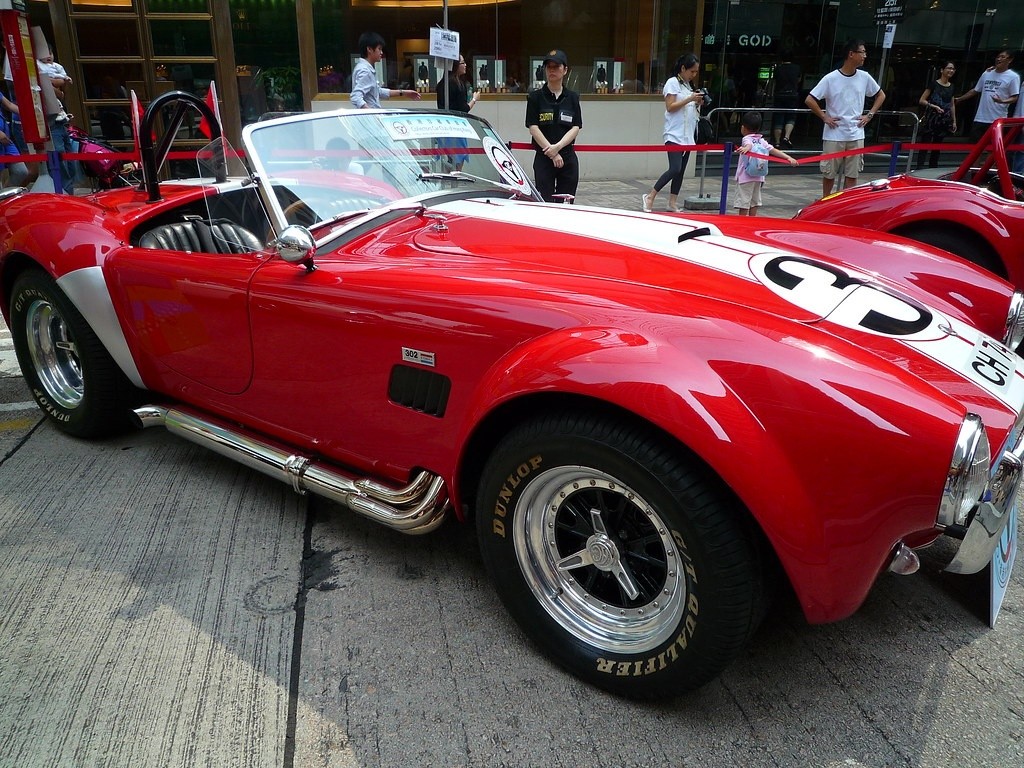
[695,87,712,105]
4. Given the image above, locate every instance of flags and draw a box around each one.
[130,89,156,163]
[199,80,228,155]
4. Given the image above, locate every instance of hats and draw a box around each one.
[542,49,568,66]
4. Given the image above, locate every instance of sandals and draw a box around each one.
[774,143,782,150]
[783,137,793,149]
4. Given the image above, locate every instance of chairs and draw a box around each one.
[307,196,383,224]
[137,220,264,254]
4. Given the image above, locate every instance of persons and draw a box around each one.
[804,37,885,199]
[642,53,704,213]
[597,64,606,83]
[616,64,644,94]
[987,66,1024,176]
[733,111,799,217]
[312,139,365,176]
[479,64,544,89]
[403,60,428,90]
[0,27,76,187]
[720,48,802,148]
[954,49,1020,185]
[350,32,422,184]
[871,53,913,129]
[525,50,582,204]
[917,59,957,169]
[436,54,480,189]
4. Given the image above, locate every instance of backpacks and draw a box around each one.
[744,137,769,177]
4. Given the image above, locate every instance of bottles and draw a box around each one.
[656,81,664,94]
[477,80,489,93]
[319,64,333,76]
[237,65,251,73]
[597,81,608,94]
[416,78,429,93]
[496,82,512,93]
[615,83,624,93]
[156,64,167,77]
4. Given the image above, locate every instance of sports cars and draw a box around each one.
[790,115,1024,297]
[0,88,1024,698]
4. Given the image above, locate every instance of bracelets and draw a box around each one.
[399,90,403,96]
[472,98,476,104]
[60,91,65,101]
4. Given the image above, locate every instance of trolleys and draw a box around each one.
[67,124,141,187]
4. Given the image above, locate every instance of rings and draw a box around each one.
[860,120,866,125]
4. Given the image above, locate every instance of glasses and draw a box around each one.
[946,67,957,72]
[853,50,867,56]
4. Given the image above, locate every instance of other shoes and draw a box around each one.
[55,111,67,121]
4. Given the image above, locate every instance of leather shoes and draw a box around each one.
[665,205,685,214]
[642,193,652,213]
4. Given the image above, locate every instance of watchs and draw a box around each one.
[867,112,874,119]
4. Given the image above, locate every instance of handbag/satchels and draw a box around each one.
[916,115,926,136]
